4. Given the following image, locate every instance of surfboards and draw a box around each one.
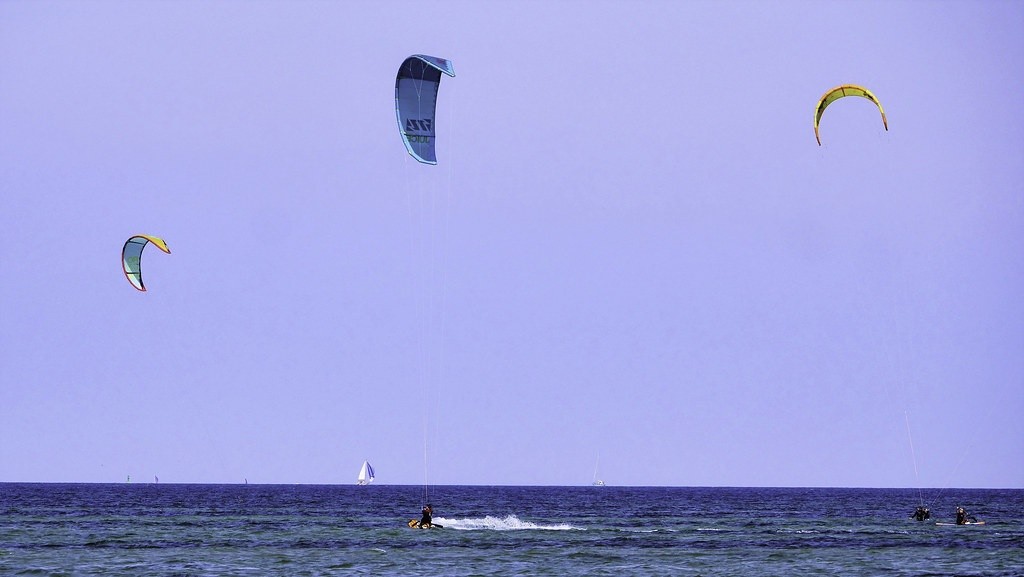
[935,521,986,526]
[409,519,444,530]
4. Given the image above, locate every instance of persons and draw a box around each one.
[957,507,968,525]
[420,504,433,529]
[912,506,926,521]
